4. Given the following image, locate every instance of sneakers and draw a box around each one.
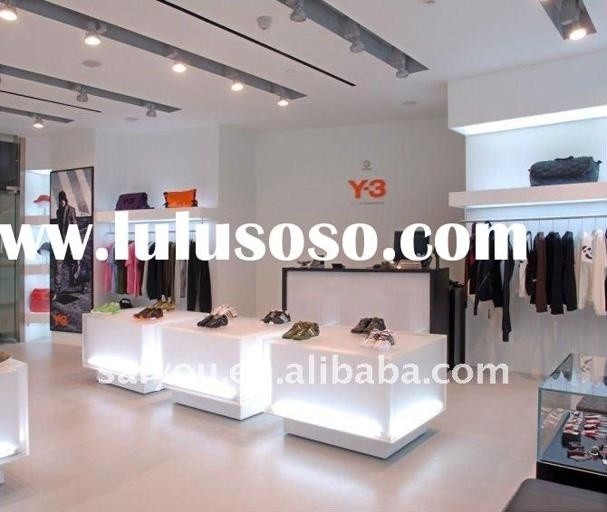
[282,321,319,340]
[362,328,397,352]
[90,302,120,316]
[197,313,228,328]
[119,298,133,310]
[258,309,291,326]
[351,317,387,333]
[149,299,176,311]
[132,306,163,320]
[212,304,237,319]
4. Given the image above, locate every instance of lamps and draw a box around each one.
[0,1,605,129]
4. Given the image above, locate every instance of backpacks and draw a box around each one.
[29,288,50,313]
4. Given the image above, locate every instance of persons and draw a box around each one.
[52,191,88,303]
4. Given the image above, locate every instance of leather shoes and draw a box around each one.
[80,285,88,295]
[52,288,62,302]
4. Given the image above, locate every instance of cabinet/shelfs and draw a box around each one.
[532,351,607,496]
[24,170,49,325]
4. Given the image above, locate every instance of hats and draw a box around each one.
[58,191,67,200]
[33,194,50,204]
[36,242,50,256]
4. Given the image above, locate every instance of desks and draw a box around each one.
[503,476,607,512]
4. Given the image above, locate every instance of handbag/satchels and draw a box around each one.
[114,192,152,208]
[68,263,89,287]
[162,189,201,208]
[529,155,601,185]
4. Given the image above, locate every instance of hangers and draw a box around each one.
[471,214,606,238]
